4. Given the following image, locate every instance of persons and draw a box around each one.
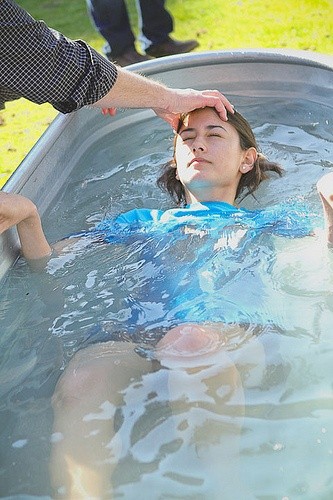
[86,0,200,67]
[0,106,333,500]
[0,0,235,133]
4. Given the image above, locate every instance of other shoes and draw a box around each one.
[106,48,150,67]
[145,37,199,58]
